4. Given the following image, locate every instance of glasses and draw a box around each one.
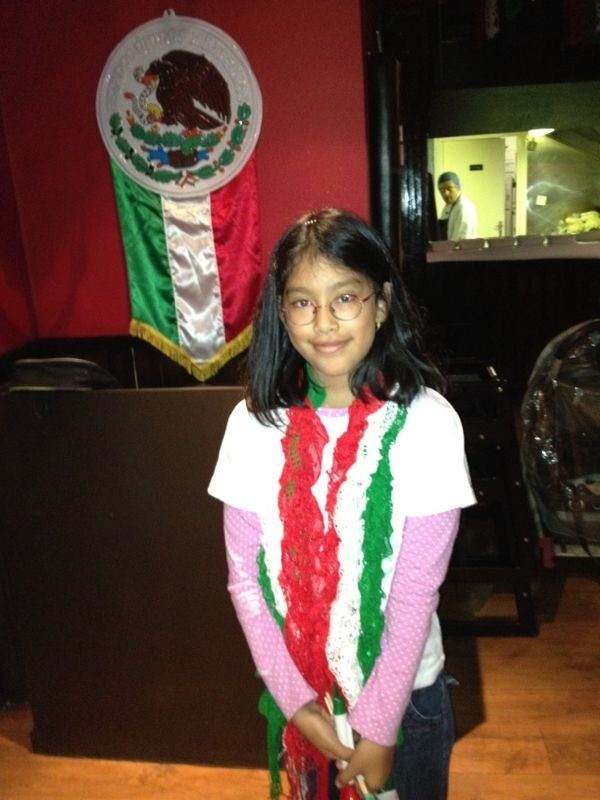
[281,290,377,325]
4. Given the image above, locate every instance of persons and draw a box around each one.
[205,208,477,800]
[437,172,477,240]
